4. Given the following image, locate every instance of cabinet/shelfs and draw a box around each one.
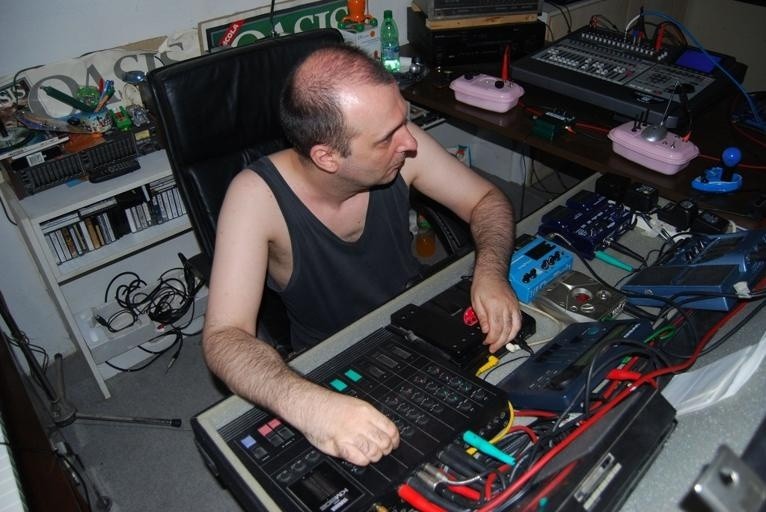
[1,149,209,399]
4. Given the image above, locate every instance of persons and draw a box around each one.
[202,43,524,468]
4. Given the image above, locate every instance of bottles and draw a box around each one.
[380,10,400,72]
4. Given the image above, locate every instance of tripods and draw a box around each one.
[0,290,182,429]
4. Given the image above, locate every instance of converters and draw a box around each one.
[696,209,729,235]
[595,172,632,204]
[624,181,658,214]
[662,199,699,228]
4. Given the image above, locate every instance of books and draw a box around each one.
[34,176,187,266]
[1,71,71,160]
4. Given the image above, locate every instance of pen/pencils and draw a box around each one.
[40,79,115,112]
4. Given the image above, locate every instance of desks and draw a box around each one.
[395,43,766,231]
[190,168,766,511]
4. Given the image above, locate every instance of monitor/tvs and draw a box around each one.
[0,327,110,512]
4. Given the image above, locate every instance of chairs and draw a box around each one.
[140,27,476,360]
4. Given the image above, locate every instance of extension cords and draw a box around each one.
[607,196,737,243]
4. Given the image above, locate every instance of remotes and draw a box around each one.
[88,158,141,184]
